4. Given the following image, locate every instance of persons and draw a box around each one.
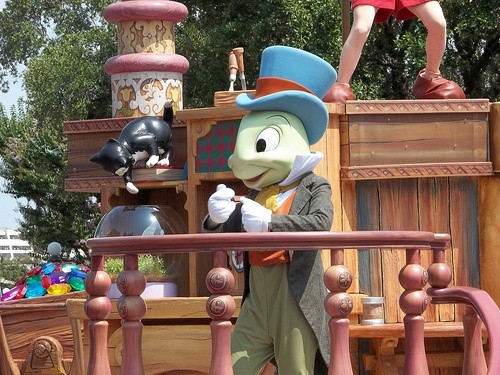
[322,0,465,104]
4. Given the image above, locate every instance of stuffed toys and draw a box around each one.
[199,45,339,375]
